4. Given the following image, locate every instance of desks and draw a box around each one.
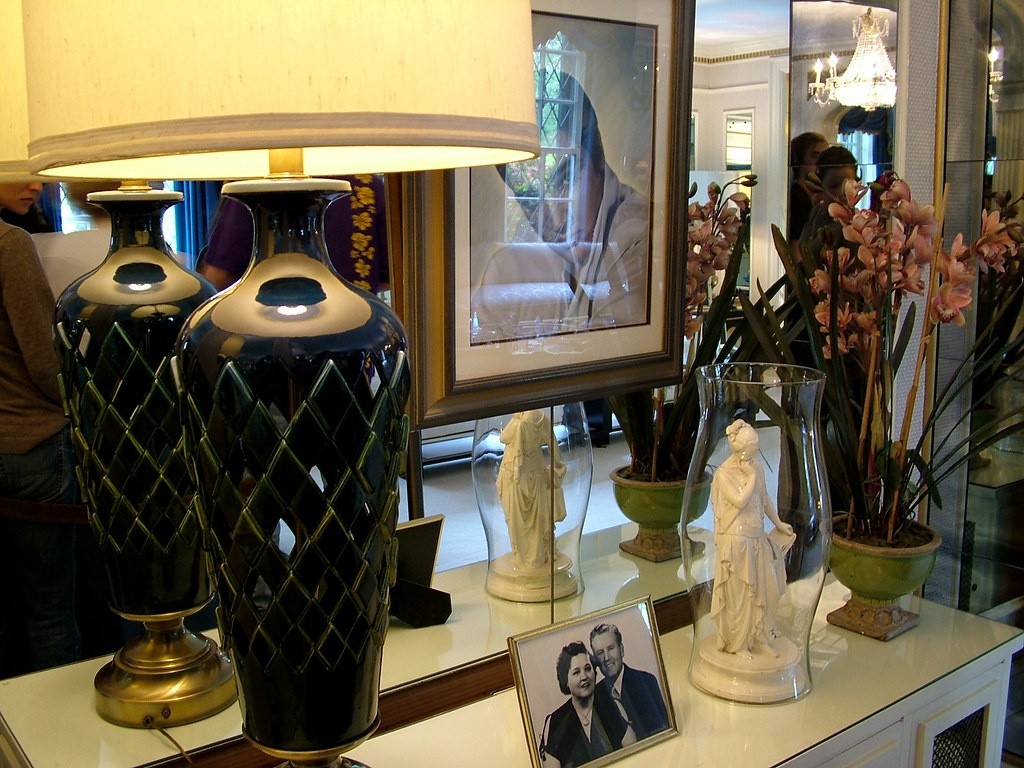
[0,517,715,768]
[374,570,1024,768]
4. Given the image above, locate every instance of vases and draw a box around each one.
[819,515,942,645]
[605,466,714,566]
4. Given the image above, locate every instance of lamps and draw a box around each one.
[988,43,1002,102]
[1,0,237,727]
[804,3,896,110]
[25,0,540,768]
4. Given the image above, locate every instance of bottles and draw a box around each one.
[471,400,596,603]
[682,362,832,708]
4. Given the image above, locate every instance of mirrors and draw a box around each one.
[0,0,951,768]
[968,0,1024,618]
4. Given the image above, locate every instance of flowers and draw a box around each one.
[628,175,760,473]
[803,162,1018,544]
[984,188,1024,219]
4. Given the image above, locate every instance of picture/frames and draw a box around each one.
[403,1,694,429]
[390,514,454,628]
[506,595,679,768]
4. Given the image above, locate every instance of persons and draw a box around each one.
[67,182,163,230]
[495,68,649,331]
[542,624,669,768]
[711,419,792,660]
[0,182,79,682]
[202,174,391,297]
[791,132,892,415]
[496,410,567,571]
